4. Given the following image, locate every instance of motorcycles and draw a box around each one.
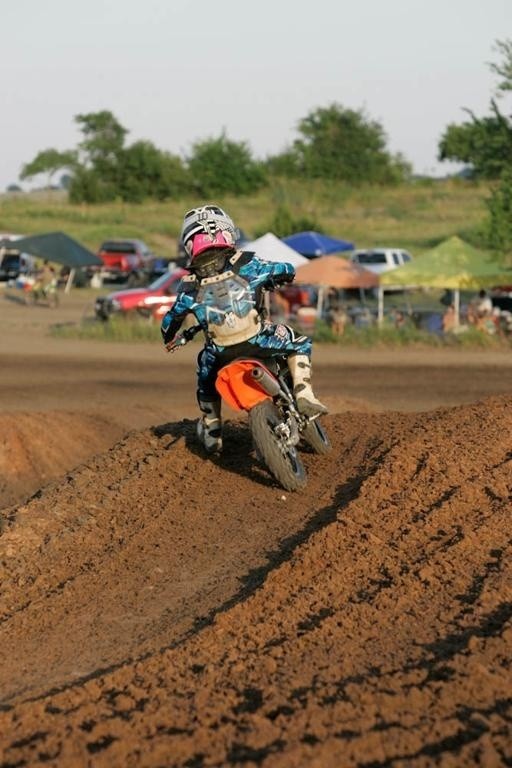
[165,277,332,495]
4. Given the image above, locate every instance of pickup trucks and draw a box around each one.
[96,240,155,283]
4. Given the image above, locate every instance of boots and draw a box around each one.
[287,352,328,419]
[195,388,224,455]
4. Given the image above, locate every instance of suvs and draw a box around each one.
[95,269,193,322]
[348,249,416,298]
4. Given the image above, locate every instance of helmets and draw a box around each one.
[181,205,237,263]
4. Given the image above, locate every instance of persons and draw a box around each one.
[160,205,324,456]
[20,257,73,303]
[327,290,510,345]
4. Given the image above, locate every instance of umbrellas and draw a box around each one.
[3,229,105,271]
[234,232,309,319]
